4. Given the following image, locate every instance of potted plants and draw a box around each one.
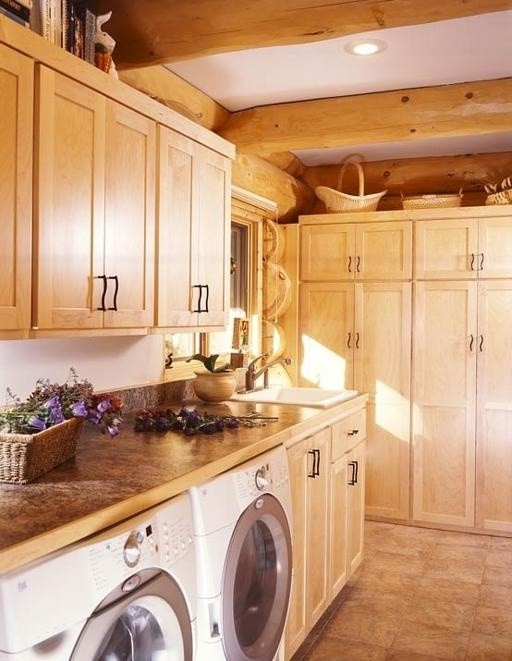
[186,351,238,404]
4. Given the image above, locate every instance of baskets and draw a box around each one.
[483,176,512,205]
[314,160,388,212]
[399,187,465,210]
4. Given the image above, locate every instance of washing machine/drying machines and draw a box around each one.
[0,493,188,661]
[189,443,300,661]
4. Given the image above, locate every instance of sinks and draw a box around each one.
[234,384,348,405]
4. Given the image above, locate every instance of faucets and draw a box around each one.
[244,353,293,390]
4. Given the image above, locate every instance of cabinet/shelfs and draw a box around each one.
[300,203,415,539]
[413,211,512,539]
[283,418,331,659]
[33,34,158,337]
[156,95,238,332]
[0,17,37,335]
[330,390,368,604]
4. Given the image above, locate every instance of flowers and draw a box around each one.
[0,363,122,438]
[135,403,279,436]
